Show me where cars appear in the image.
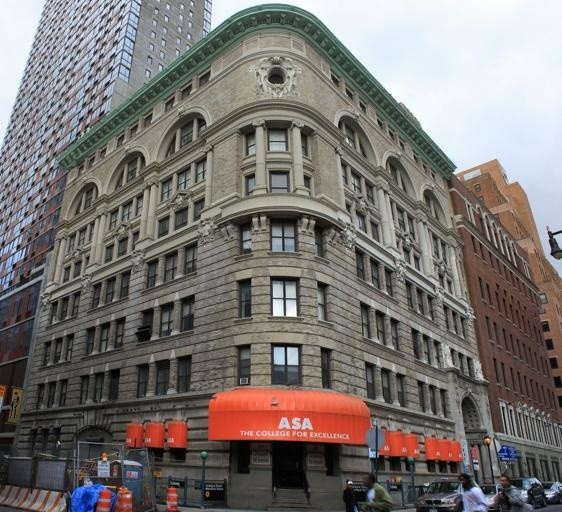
[415,477,562,512]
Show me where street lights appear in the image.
[483,435,495,484]
[545,226,562,260]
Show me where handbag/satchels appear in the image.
[520,503,533,512]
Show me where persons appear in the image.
[343,481,360,512]
[362,473,393,512]
[458,473,490,512]
[491,475,525,512]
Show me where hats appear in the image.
[347,481,353,485]
